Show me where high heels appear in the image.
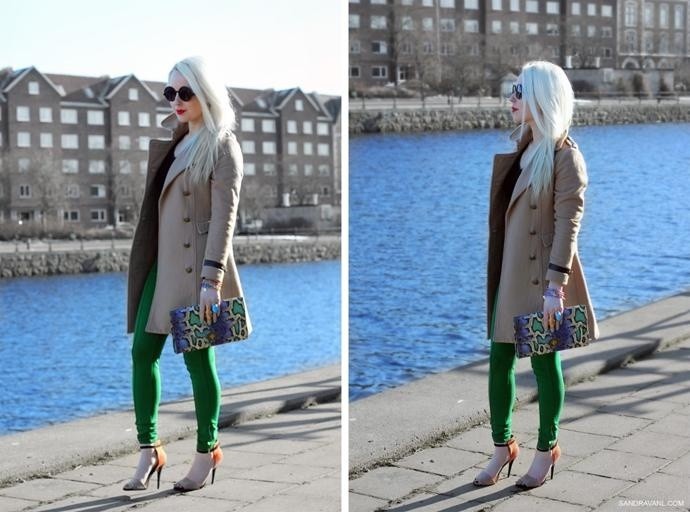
[515,439,561,491]
[173,440,224,492]
[472,435,519,487]
[123,439,168,490]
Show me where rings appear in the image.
[554,309,563,323]
[212,303,222,314]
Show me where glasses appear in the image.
[512,84,523,99]
[163,86,195,102]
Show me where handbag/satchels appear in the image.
[168,297,252,355]
[513,305,590,358]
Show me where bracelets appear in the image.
[196,280,222,289]
[542,285,568,298]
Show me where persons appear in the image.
[469,59,602,490]
[123,52,257,495]
